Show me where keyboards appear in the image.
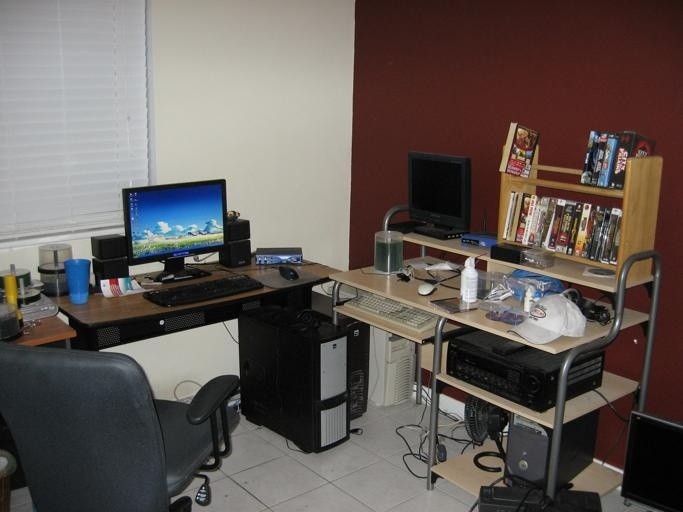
[143,273,264,308]
[343,292,438,334]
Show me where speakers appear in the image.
[90,234,130,282]
[219,216,252,269]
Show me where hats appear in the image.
[510,294,585,345]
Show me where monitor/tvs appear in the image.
[621,409,683,511]
[408,149,471,241]
[121,177,227,282]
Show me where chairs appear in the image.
[0,342,240,512]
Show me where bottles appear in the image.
[374,231,404,272]
[523,288,534,312]
[460,256,477,304]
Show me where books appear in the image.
[21,293,58,321]
[499,122,656,266]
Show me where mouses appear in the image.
[280,266,300,281]
[416,281,436,297]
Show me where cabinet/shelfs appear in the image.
[497,145,663,288]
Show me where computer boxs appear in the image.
[503,410,600,488]
[238,312,350,453]
[369,325,417,407]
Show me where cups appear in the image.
[64,259,91,305]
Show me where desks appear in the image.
[0,316,78,350]
[329,204,662,507]
[46,258,341,427]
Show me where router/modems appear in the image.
[461,232,496,249]
